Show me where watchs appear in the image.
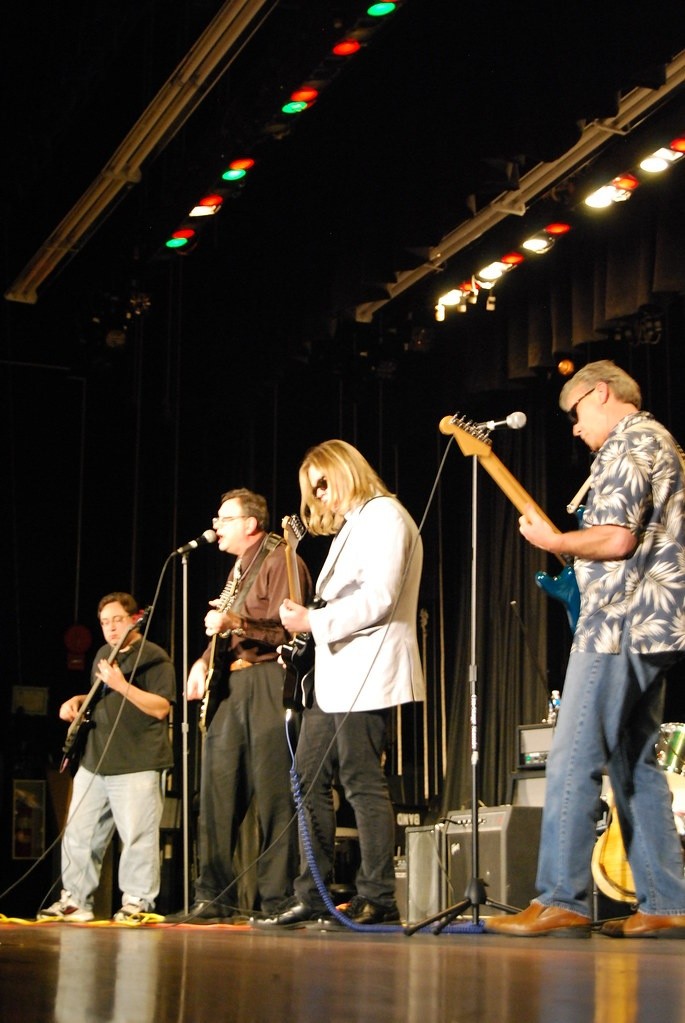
[232,616,244,636]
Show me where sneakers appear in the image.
[36,889,95,923]
[113,899,154,923]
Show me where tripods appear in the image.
[402,451,522,937]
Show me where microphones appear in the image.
[171,530,217,556]
[475,412,526,430]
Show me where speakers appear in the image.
[401,770,633,926]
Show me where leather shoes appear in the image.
[248,895,333,930]
[598,909,685,939]
[484,900,593,939]
[317,895,401,932]
[166,900,240,925]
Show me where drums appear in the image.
[655,721,685,816]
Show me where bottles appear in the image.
[547,690,560,724]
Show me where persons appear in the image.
[483,359,685,943]
[36,592,175,922]
[279,440,424,922]
[176,489,313,921]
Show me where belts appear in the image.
[223,659,261,671]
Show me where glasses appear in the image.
[99,615,129,626]
[212,514,250,525]
[567,380,611,425]
[311,475,327,498]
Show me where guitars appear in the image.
[199,576,239,729]
[280,511,328,712]
[57,603,154,779]
[439,410,588,636]
